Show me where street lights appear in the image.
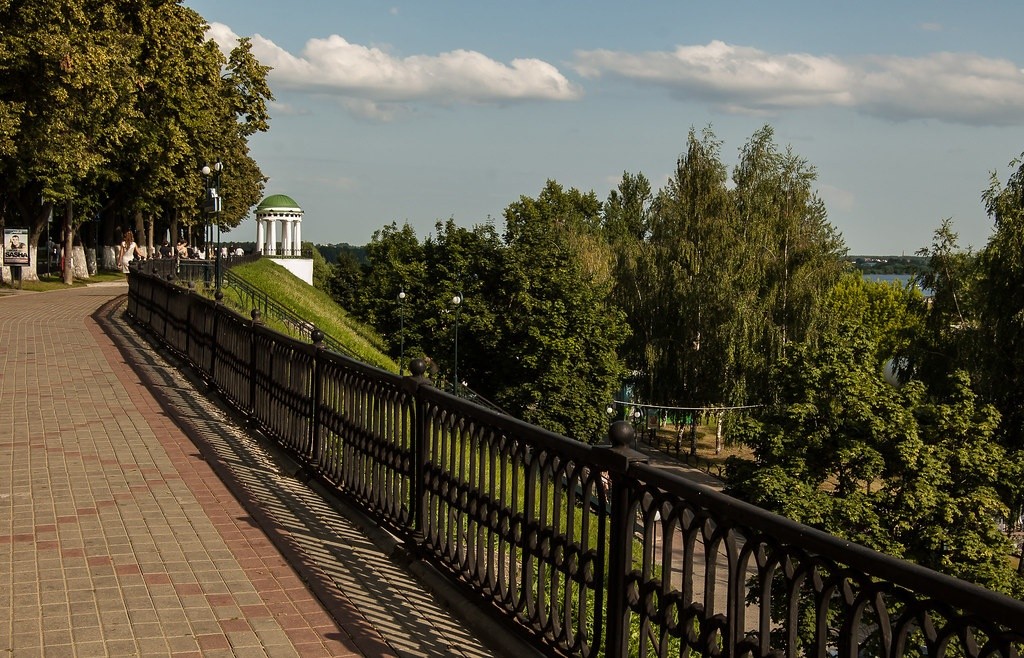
[203,165,212,287]
[451,296,464,399]
[214,161,225,293]
[635,411,640,453]
[607,407,613,445]
[399,291,406,377]
